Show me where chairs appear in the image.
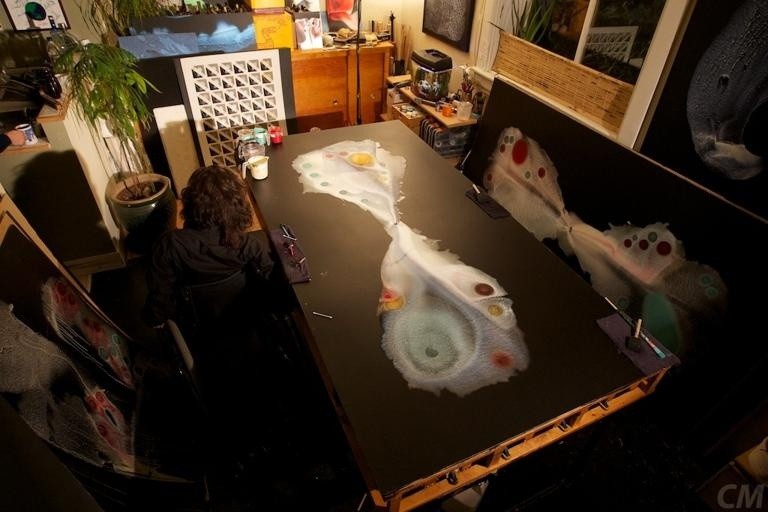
[185,270,254,335]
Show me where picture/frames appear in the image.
[420,0,475,52]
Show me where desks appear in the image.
[237,120,682,511]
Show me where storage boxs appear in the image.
[409,49,452,102]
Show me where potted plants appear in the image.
[41,24,180,253]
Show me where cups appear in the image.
[442,102,455,116]
[456,100,473,120]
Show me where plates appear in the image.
[344,150,387,173]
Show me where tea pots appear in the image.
[234,128,256,146]
[242,155,270,180]
[237,141,265,162]
[254,127,271,147]
[267,120,282,144]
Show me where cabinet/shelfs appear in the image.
[291,43,394,133]
[381,76,480,167]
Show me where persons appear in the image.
[122,164,274,336]
